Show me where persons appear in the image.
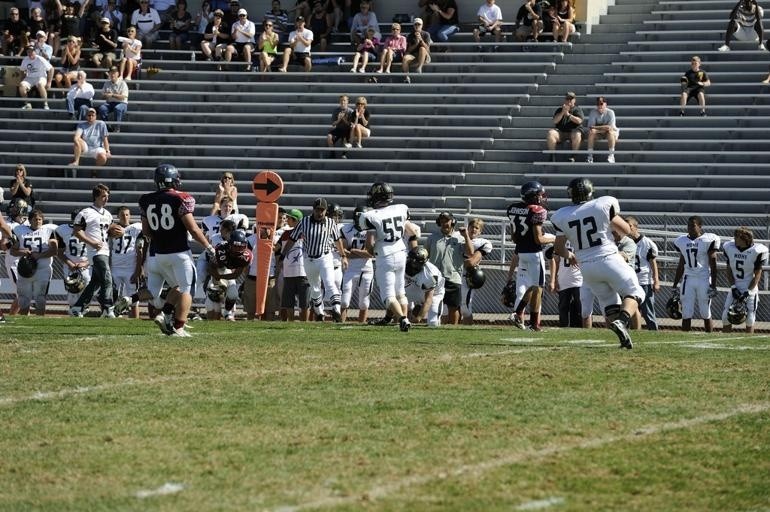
[325,94,352,147]
[553,176,649,349]
[584,97,619,163]
[211,169,240,218]
[176,0,580,73]
[716,0,767,53]
[0,0,179,134]
[347,97,373,148]
[0,180,769,334]
[67,107,113,168]
[137,161,222,338]
[545,91,584,162]
[7,163,37,217]
[678,55,711,118]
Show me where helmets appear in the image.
[17,255,38,278]
[7,198,28,221]
[228,232,247,258]
[406,246,430,277]
[284,209,303,221]
[667,295,683,320]
[465,266,487,289]
[65,273,84,293]
[568,178,595,206]
[498,281,517,307]
[520,181,545,206]
[325,202,344,223]
[727,300,747,324]
[154,165,181,190]
[368,182,393,210]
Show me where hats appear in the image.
[296,16,304,22]
[238,8,247,15]
[565,92,576,98]
[440,211,451,220]
[36,30,46,38]
[414,18,422,25]
[88,108,95,113]
[101,17,109,24]
[313,198,328,210]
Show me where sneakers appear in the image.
[376,315,411,331]
[717,45,730,51]
[67,297,238,339]
[610,320,632,350]
[475,45,503,52]
[22,103,33,109]
[514,317,545,332]
[316,309,342,322]
[606,155,614,162]
[758,44,767,50]
[43,103,49,109]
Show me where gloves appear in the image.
[733,287,750,301]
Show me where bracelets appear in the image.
[106,151,110,153]
[567,113,572,123]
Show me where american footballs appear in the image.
[108,221,123,237]
